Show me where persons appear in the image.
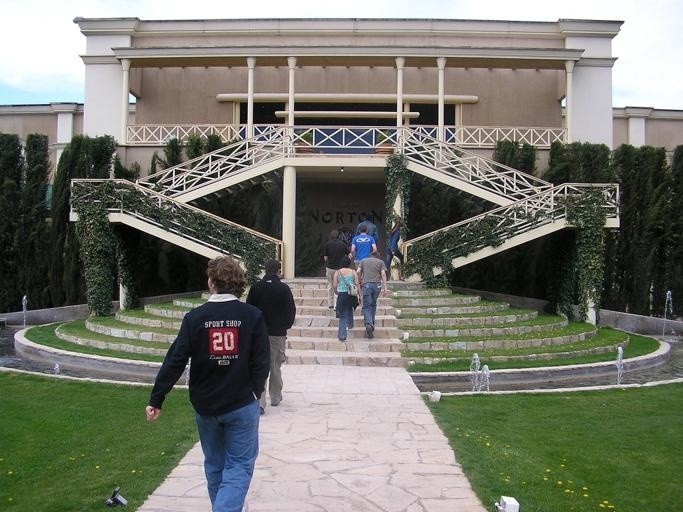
[322,211,409,342]
[246,259,298,413]
[144,257,273,510]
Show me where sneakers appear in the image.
[366,325,374,338]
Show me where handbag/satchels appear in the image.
[347,284,358,296]
